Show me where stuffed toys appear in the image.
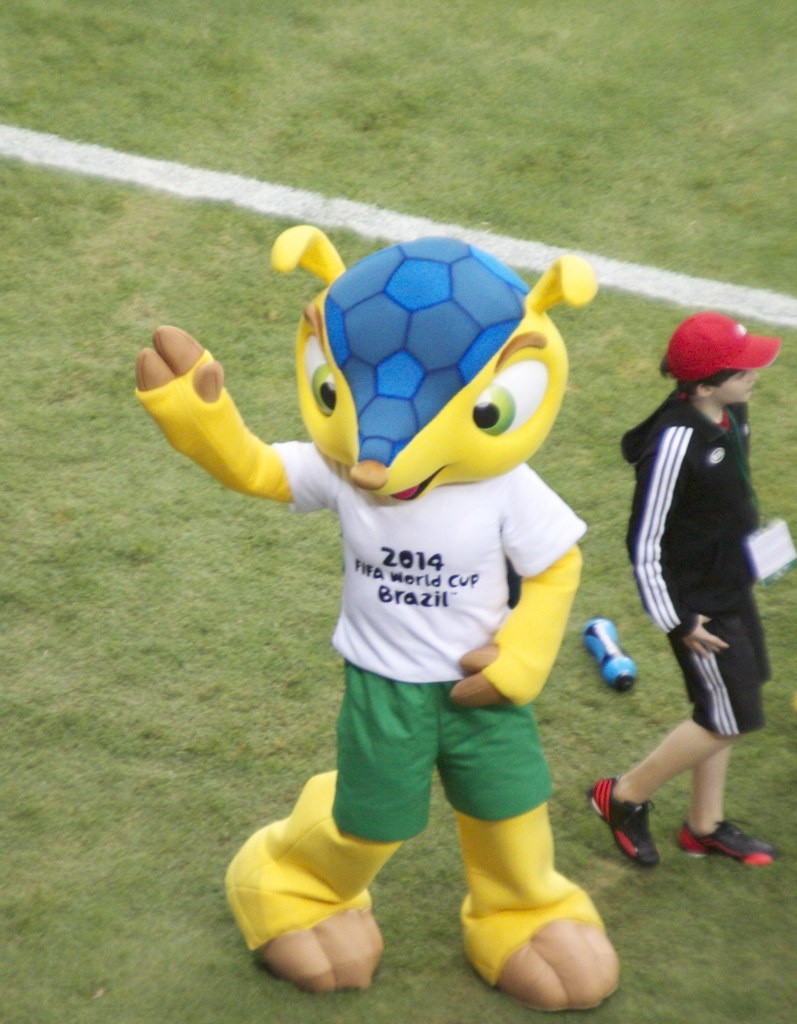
[131,225,619,1011]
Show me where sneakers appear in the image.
[586,774,661,867]
[676,819,781,866]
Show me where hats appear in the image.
[667,311,782,382]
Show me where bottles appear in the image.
[581,617,636,692]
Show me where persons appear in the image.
[583,312,783,870]
